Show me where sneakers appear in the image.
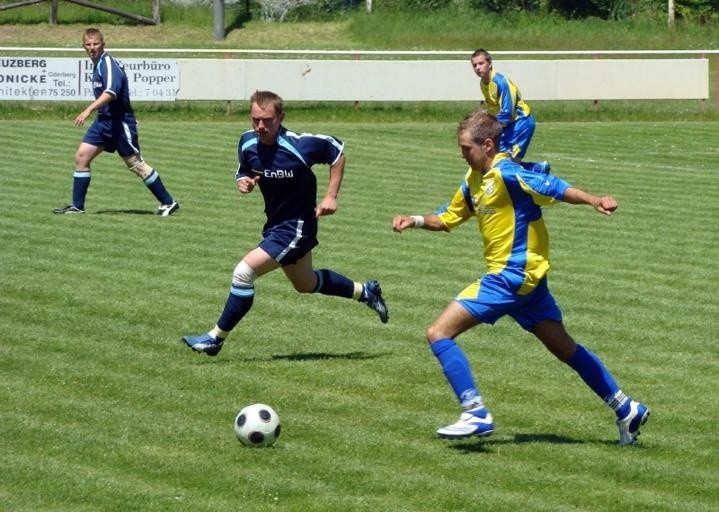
[53,205,84,214]
[182,333,224,355]
[436,405,494,436]
[364,280,387,323]
[156,200,178,216]
[616,400,650,447]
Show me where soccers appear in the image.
[233,404,280,448]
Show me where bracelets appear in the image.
[410,214,427,230]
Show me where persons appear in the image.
[52,27,181,219]
[388,112,652,449]
[181,88,389,355]
[471,46,536,164]
